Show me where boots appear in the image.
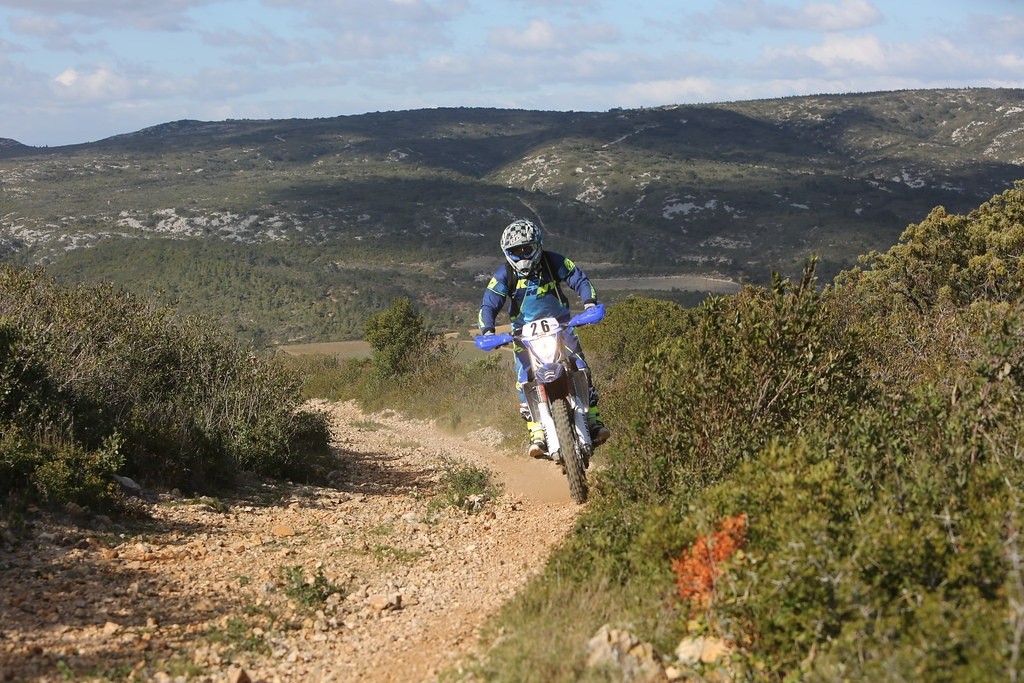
[587,406,610,442]
[527,421,548,459]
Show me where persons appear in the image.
[477,220,611,459]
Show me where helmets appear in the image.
[500,220,543,277]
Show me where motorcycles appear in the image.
[474,304,607,505]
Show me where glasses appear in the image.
[504,242,537,260]
